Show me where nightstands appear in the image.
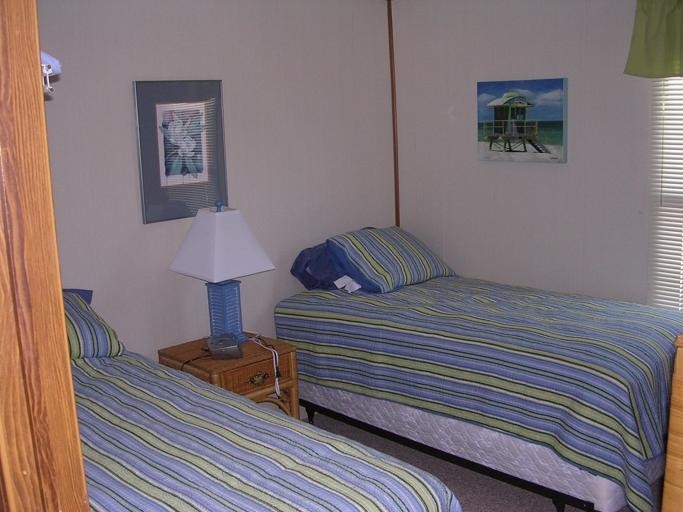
[154,327,304,422]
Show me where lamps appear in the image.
[165,199,278,352]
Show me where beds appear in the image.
[48,281,476,512]
[261,263,681,511]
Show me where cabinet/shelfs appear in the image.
[652,329,681,511]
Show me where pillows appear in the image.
[324,224,457,296]
[57,286,132,365]
[285,224,382,293]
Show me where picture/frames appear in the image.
[130,76,231,227]
